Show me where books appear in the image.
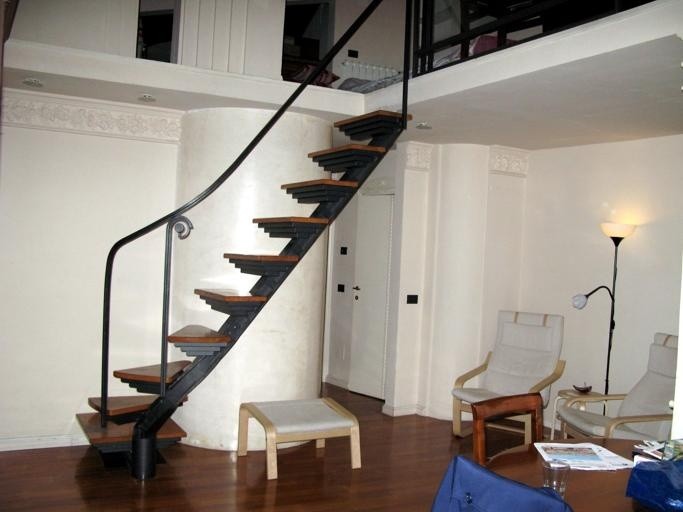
[532,438,682,472]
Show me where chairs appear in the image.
[469,392,544,467]
[556,331,677,442]
[451,311,567,445]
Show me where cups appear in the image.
[540,460,571,500]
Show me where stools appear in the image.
[236,397,363,481]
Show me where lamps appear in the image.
[571,223,636,416]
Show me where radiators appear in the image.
[342,61,399,83]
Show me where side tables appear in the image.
[549,388,609,442]
